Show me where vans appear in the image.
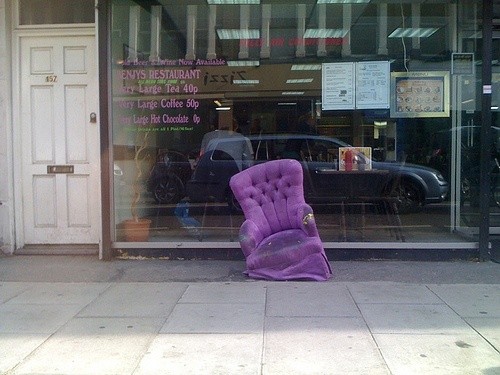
[189,133,447,215]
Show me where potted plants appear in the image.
[122,52,180,242]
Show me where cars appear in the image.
[142,143,203,204]
[114,164,127,191]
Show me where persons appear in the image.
[297,110,320,136]
[200,117,255,210]
[345,148,366,172]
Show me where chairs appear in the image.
[148,149,240,242]
[230,159,333,281]
[360,149,407,243]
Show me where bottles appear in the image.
[345,148,352,172]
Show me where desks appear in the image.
[315,166,389,242]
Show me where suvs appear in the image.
[433,124,499,206]
